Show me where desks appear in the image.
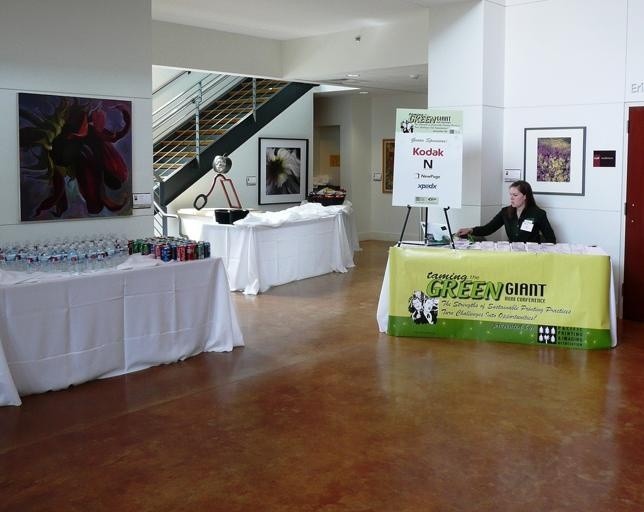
[0,254,244,410]
[376,241,617,351]
[180,206,363,295]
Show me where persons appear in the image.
[455,181,556,245]
[411,291,437,325]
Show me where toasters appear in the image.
[1,233,130,276]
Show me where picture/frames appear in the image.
[382,138,395,194]
[523,128,586,196]
[258,137,309,205]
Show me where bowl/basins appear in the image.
[451,239,591,253]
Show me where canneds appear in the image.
[128,235,211,262]
